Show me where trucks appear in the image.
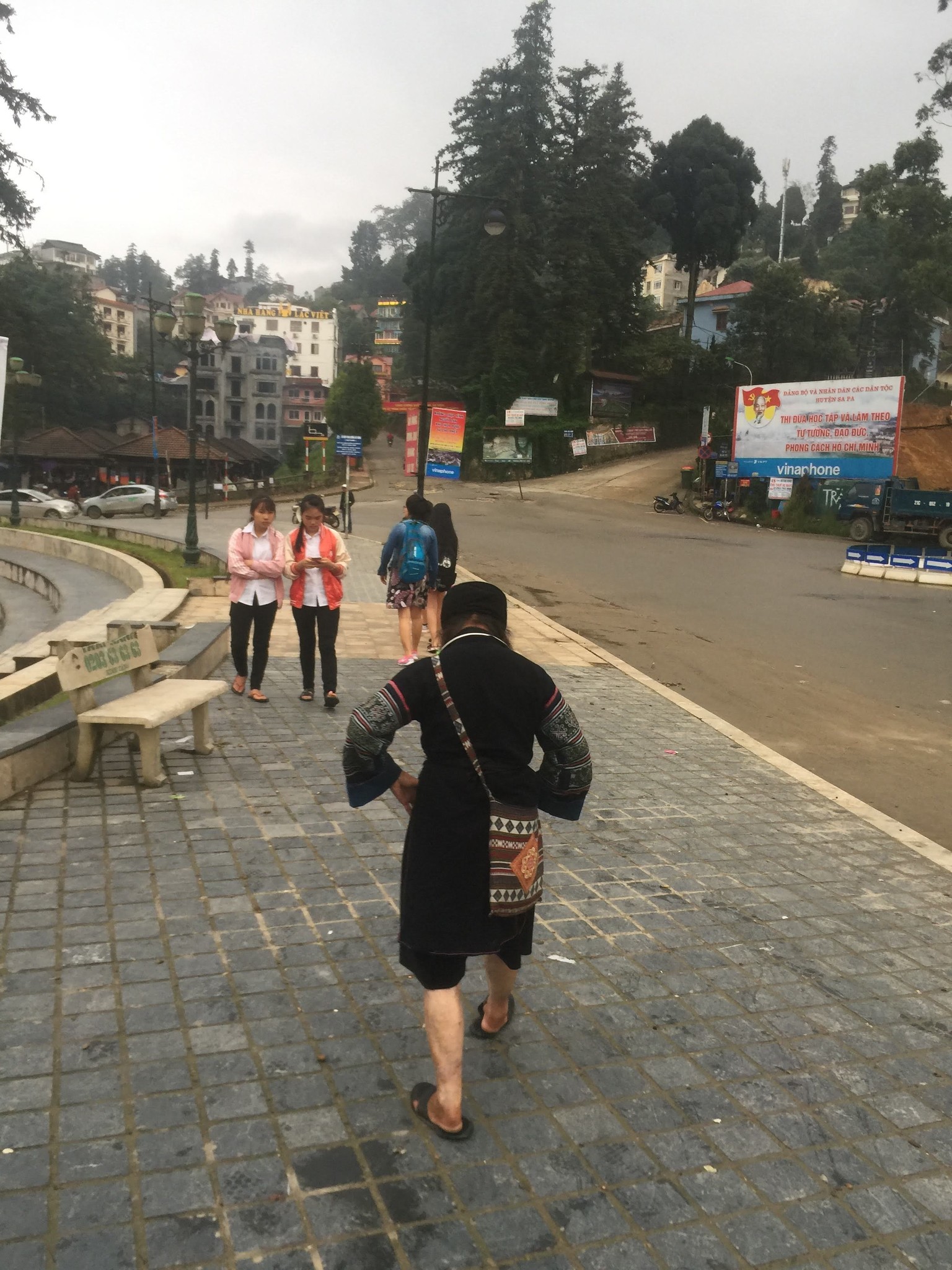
[835,475,952,551]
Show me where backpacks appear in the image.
[399,521,426,582]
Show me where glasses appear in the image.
[403,506,407,509]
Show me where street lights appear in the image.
[778,157,791,264]
[725,356,753,386]
[407,157,512,496]
[154,292,238,565]
[141,280,178,520]
[4,357,42,526]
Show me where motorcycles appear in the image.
[652,492,685,514]
[702,498,731,522]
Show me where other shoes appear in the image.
[348,531,352,533]
[341,530,345,532]
[426,643,441,654]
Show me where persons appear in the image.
[748,394,771,428]
[283,494,352,707]
[68,485,82,509]
[386,433,394,445]
[421,500,457,653]
[341,582,593,1142]
[228,495,285,703]
[377,493,438,665]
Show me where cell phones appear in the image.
[312,557,322,563]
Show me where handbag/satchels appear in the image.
[488,800,544,916]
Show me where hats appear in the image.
[342,484,347,488]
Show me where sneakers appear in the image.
[421,623,430,633]
[411,650,418,660]
[398,655,414,665]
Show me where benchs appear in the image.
[56,622,228,783]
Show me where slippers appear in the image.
[409,1082,473,1139]
[299,688,315,701]
[232,674,248,695]
[248,693,269,703]
[324,694,339,708]
[469,992,514,1039]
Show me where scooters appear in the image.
[64,490,80,503]
[388,437,394,447]
[292,501,302,525]
[320,494,340,528]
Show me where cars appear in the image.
[82,484,179,520]
[0,488,80,520]
